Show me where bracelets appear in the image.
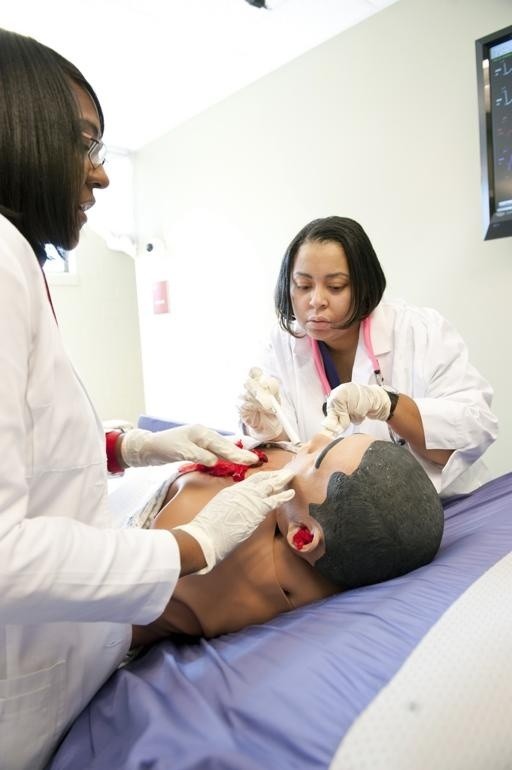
[104,424,127,474]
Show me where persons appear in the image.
[0,24,318,770]
[97,431,445,647]
[233,215,499,500]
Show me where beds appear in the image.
[49,417,511,769]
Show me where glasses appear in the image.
[80,129,105,170]
